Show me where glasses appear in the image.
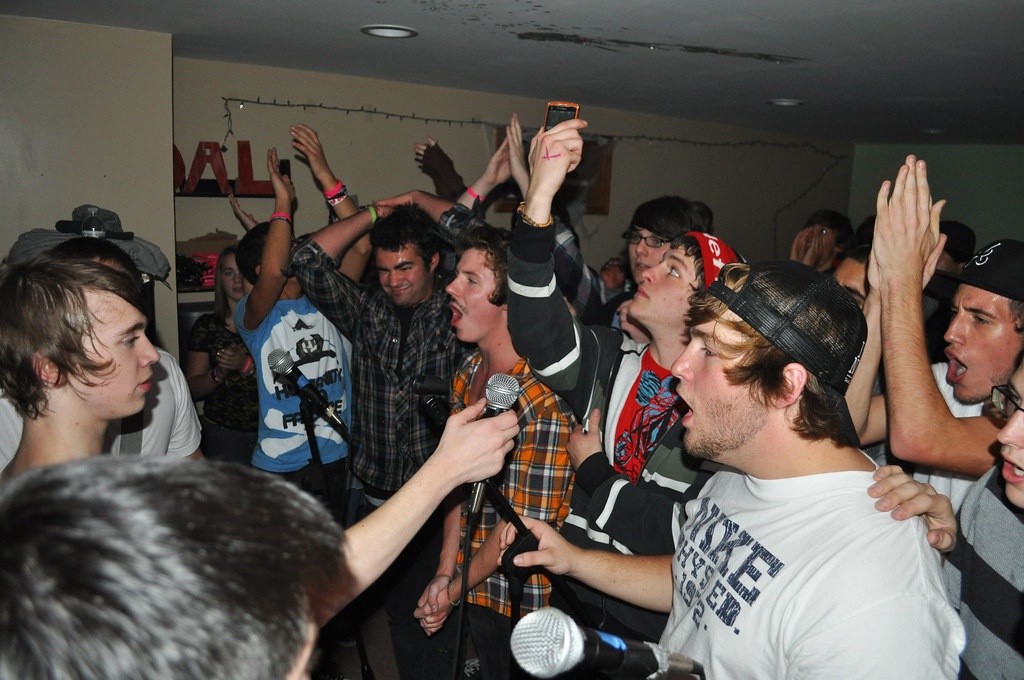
[991,384,1023,418]
[621,231,673,248]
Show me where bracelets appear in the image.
[245,367,256,377]
[210,367,224,383]
[322,179,342,198]
[447,577,461,606]
[367,206,376,224]
[328,195,347,207]
[466,186,486,202]
[208,369,217,384]
[329,184,347,199]
[270,212,291,223]
[516,202,553,227]
[242,356,253,373]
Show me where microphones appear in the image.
[269,347,348,434]
[510,606,704,678]
[468,373,520,527]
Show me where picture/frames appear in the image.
[490,124,613,216]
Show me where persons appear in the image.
[0,111,1024,678]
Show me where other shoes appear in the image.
[338,631,357,648]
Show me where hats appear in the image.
[938,219,976,258]
[941,239,1024,299]
[685,231,742,289]
[707,258,868,446]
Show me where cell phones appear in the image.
[543,102,580,134]
